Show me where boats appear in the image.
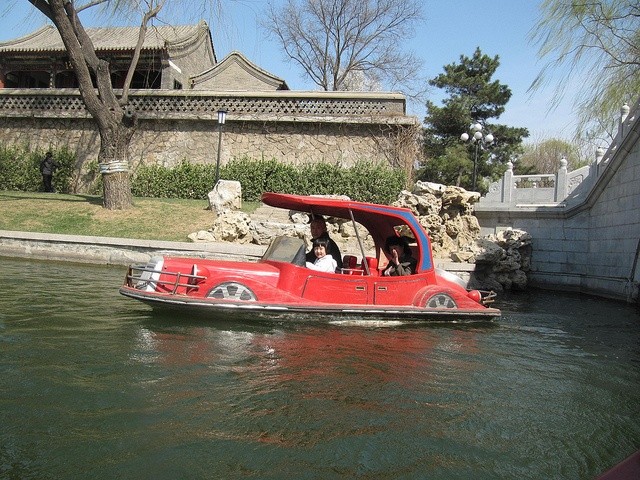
[119,192,502,318]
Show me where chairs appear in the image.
[409,246,419,261]
[336,255,361,274]
[353,256,379,276]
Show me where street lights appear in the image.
[216,108,228,184]
[460,123,494,191]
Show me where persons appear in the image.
[382,235,417,276]
[306,238,338,273]
[41,152,57,192]
[305,217,343,273]
